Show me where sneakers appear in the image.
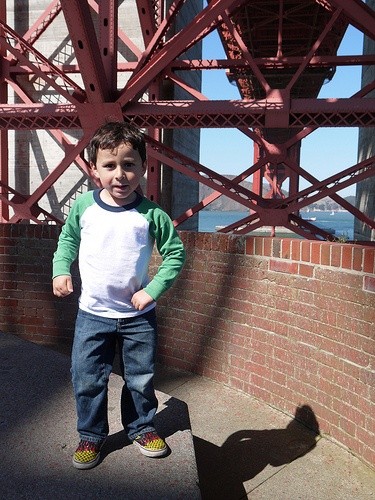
[72,436,108,469]
[132,431,168,457]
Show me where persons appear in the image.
[53,122,186,470]
